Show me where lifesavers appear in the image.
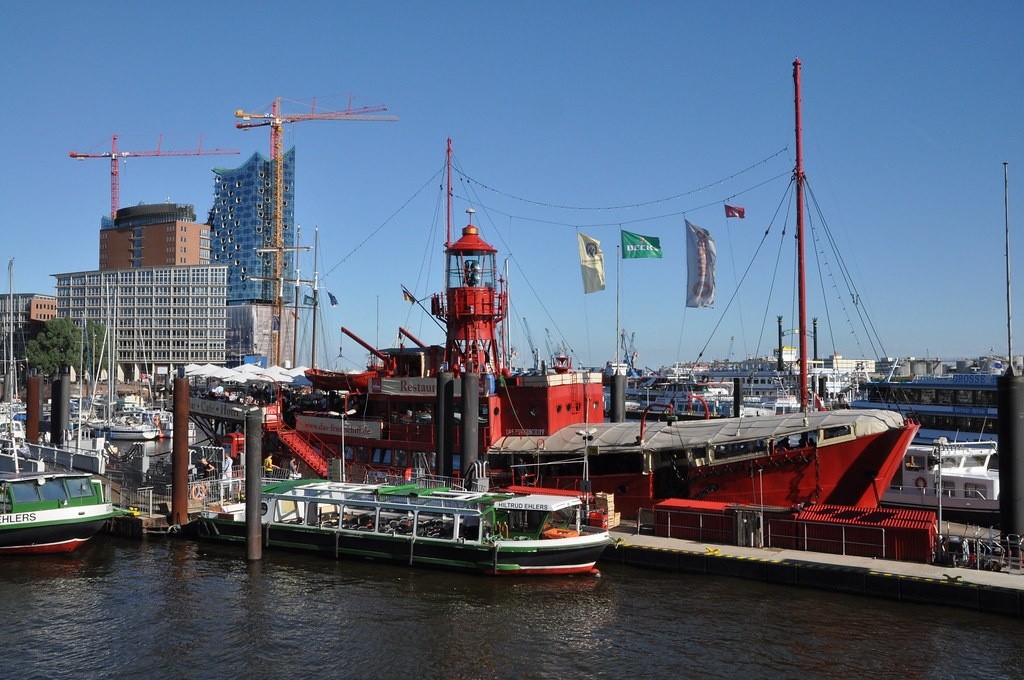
[915,477,927,490]
[155,461,165,475]
[191,484,207,500]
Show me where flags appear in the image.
[621,230,662,259]
[725,205,745,219]
[685,219,716,310]
[578,232,605,295]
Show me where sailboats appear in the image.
[0,58,1003,579]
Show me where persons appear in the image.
[225,452,233,500]
[264,454,273,472]
[289,457,297,474]
[207,377,340,412]
[201,457,214,478]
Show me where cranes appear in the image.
[233,92,400,363]
[68,131,240,220]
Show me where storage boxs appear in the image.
[579,492,622,532]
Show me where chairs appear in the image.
[323,511,444,537]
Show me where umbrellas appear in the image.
[170,362,362,386]
